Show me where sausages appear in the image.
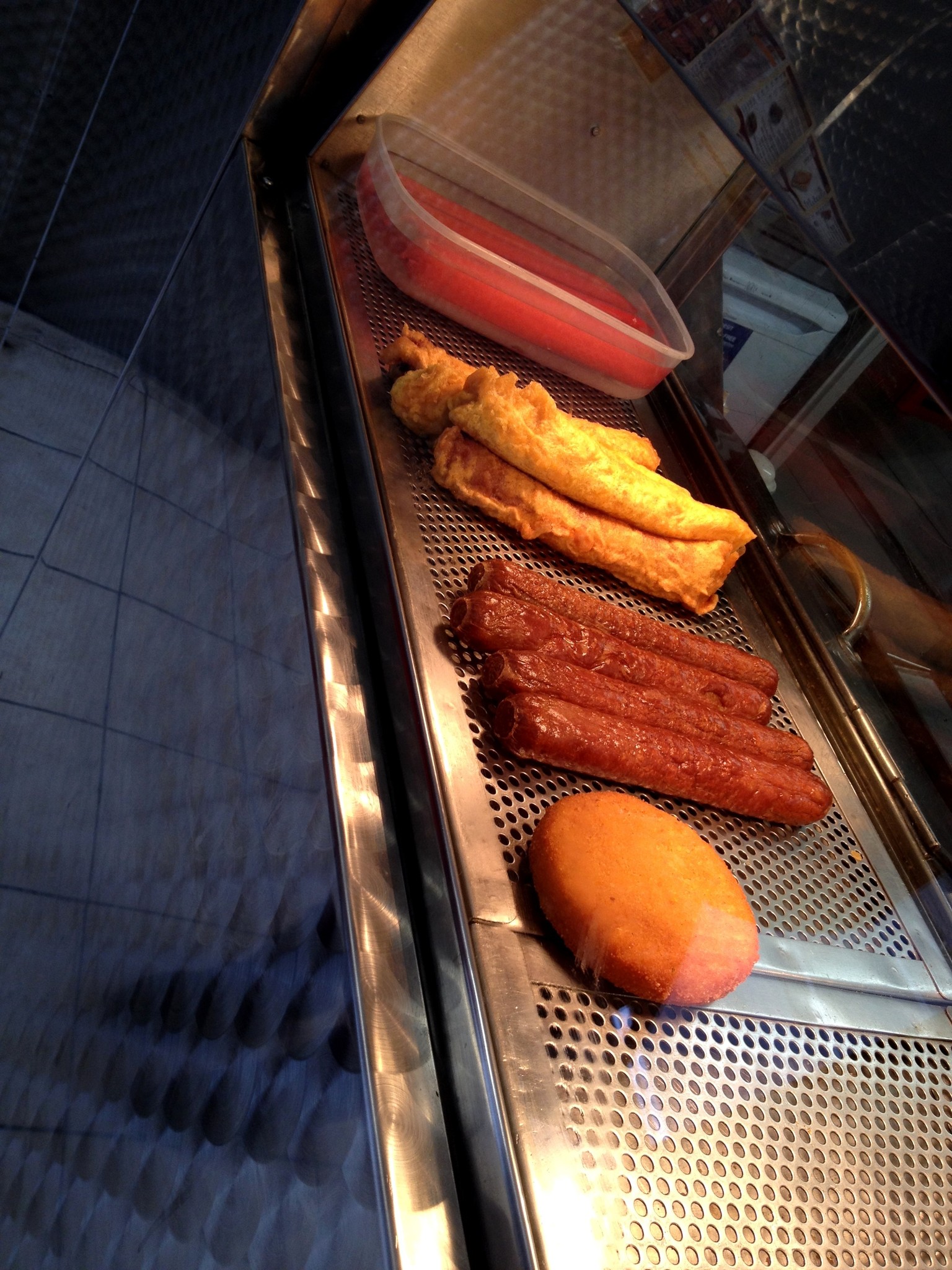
[448,557,835,829]
[374,162,670,386]
